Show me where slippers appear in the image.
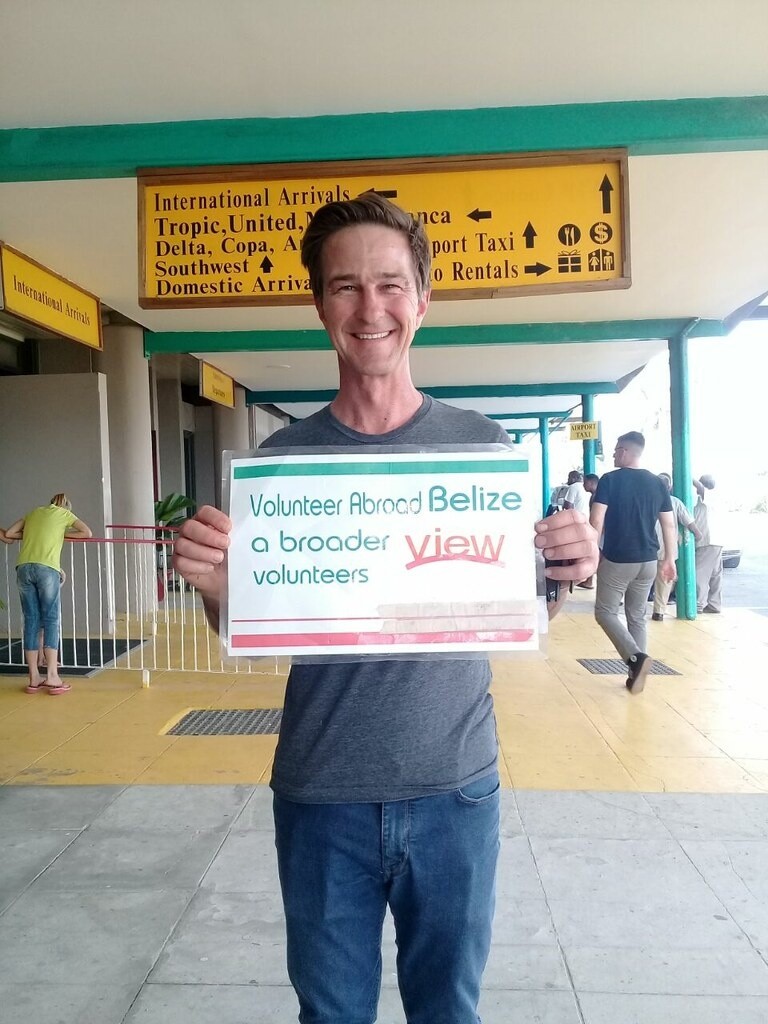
[577,582,594,589]
[25,680,47,693]
[49,681,73,695]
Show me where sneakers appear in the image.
[625,652,651,696]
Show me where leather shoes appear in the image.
[651,611,664,621]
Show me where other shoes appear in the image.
[696,605,722,614]
[666,600,676,605]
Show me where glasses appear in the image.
[545,504,574,603]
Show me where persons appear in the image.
[562,471,593,523]
[588,432,677,694]
[0,493,93,695]
[693,474,725,614]
[576,474,604,589]
[647,473,703,621]
[172,191,599,1024]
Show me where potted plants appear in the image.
[153,492,199,602]
